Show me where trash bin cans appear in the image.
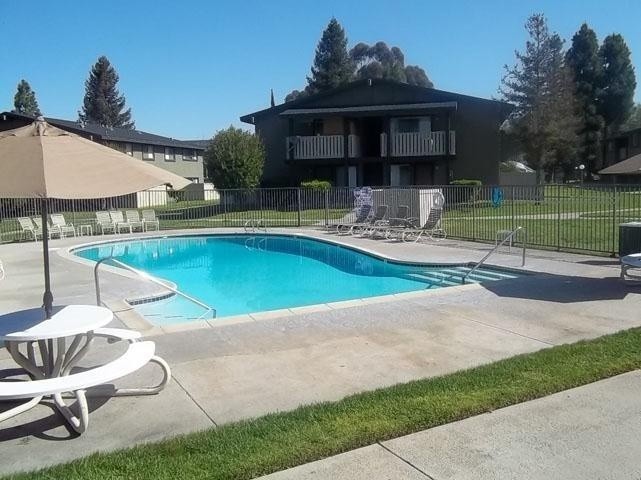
[619,222,641,257]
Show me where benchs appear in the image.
[0,304,172,435]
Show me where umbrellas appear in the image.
[0,114,195,319]
[595,150,641,176]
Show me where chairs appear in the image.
[94,208,161,234]
[325,203,448,243]
[17,213,76,242]
[620,252,641,286]
